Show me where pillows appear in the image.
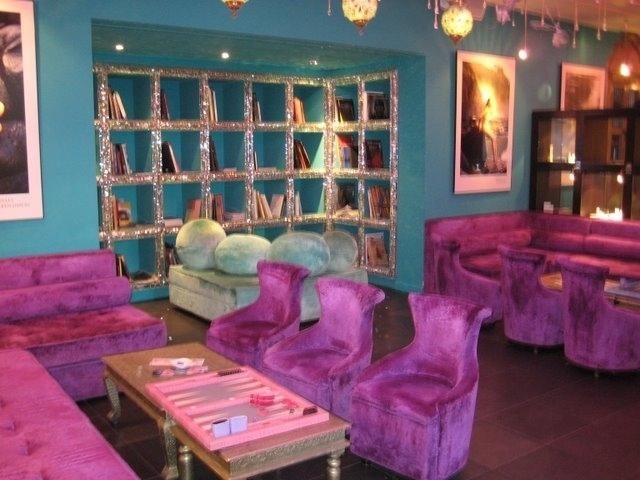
[175,218,360,282]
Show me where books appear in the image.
[364,91,386,266]
[337,97,358,217]
[109,91,130,282]
[294,98,311,218]
[208,88,243,223]
[251,92,284,221]
[159,89,201,272]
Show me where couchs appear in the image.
[425,210,639,294]
[0,250,167,480]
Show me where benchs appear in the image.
[161,226,372,329]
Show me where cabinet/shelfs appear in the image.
[529,109,639,222]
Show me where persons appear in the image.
[477,97,502,172]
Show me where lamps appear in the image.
[338,0,474,46]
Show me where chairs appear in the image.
[432,231,639,376]
